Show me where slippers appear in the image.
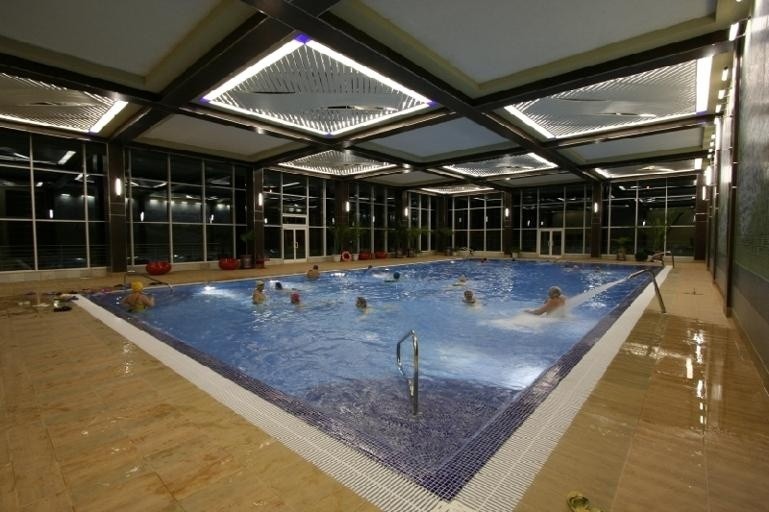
[567,491,605,512]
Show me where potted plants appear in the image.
[510,249,521,257]
[240,232,254,269]
[642,214,668,261]
[332,219,369,262]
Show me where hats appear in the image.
[393,271,400,279]
[291,292,300,300]
[256,280,265,287]
[313,265,318,269]
[358,296,367,306]
[463,291,473,295]
[131,282,143,291]
[549,286,561,296]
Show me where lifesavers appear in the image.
[341,251,353,262]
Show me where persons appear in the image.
[120,280,155,314]
[454,274,469,286]
[461,289,476,303]
[304,264,321,280]
[355,296,369,314]
[290,292,301,304]
[274,282,282,290]
[252,279,266,304]
[524,286,567,318]
